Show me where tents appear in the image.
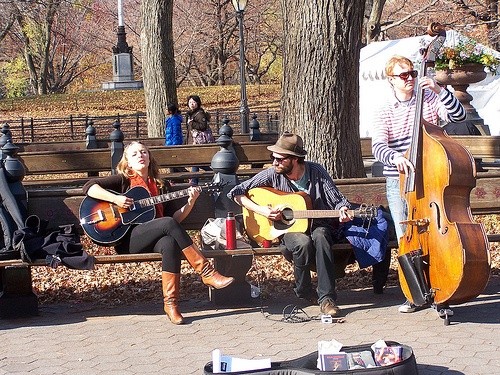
[360,28,500,137]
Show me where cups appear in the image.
[263,240,272,248]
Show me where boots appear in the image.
[162,271,185,324]
[182,242,235,290]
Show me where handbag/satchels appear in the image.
[200,216,243,247]
[190,111,215,144]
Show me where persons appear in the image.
[164,103,189,183]
[83,140,235,324]
[186,95,217,184]
[227,131,353,317]
[371,56,467,313]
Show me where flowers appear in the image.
[422,35,500,76]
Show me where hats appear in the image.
[267,130,308,158]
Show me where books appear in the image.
[320,347,401,370]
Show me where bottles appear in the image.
[226,212,237,250]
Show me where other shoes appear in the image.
[320,299,340,316]
[398,300,419,313]
[308,289,318,305]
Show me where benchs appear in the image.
[0,138,500,304]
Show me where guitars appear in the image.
[79,182,224,248]
[242,186,378,248]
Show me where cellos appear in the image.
[397,21,493,327]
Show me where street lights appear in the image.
[231,0,249,133]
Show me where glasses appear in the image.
[270,154,291,162]
[165,108,168,111]
[388,70,418,79]
